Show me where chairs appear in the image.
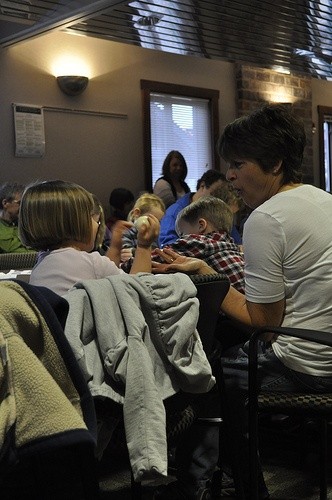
[0,253,38,273]
[239,326,332,500]
[167,273,231,500]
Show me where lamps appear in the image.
[57,54,88,97]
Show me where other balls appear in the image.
[135,216,150,232]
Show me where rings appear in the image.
[168,257,175,264]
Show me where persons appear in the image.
[150,103,332,500]
[0,151,255,347]
